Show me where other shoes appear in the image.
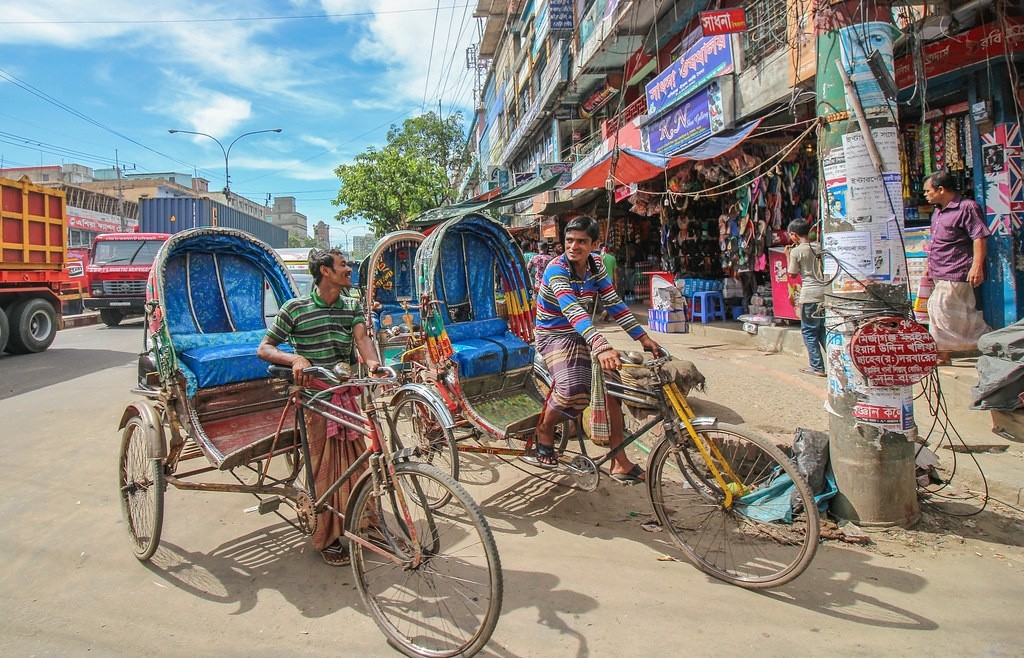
[599,318,609,324]
[936,357,953,366]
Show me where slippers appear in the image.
[800,368,825,377]
[659,142,820,277]
[369,527,403,551]
[536,434,558,465]
[613,463,646,480]
[321,538,350,566]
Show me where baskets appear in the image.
[616,352,693,409]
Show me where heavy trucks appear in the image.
[81,194,289,324]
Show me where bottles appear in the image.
[624,261,653,304]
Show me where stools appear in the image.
[691,291,726,326]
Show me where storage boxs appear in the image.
[648,309,686,334]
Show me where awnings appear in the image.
[406,113,764,228]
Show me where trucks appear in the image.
[0,174,72,357]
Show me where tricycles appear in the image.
[387,211,822,591]
[119,225,506,656]
[360,231,428,380]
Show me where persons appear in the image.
[534,215,665,484]
[923,172,996,366]
[737,265,760,315]
[256,246,395,567]
[519,240,619,324]
[784,218,828,377]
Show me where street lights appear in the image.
[166,128,283,190]
[326,225,365,253]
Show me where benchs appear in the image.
[367,229,426,366]
[411,208,539,403]
[134,222,304,424]
